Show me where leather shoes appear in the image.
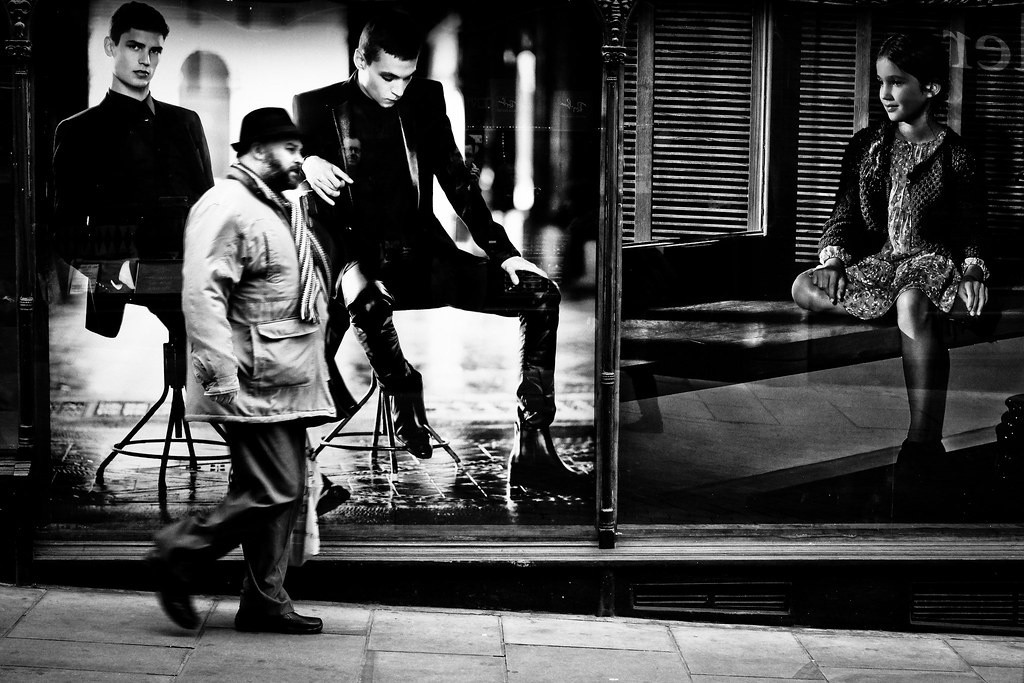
[142,548,203,630]
[389,381,433,459]
[509,421,583,498]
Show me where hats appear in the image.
[231,107,307,152]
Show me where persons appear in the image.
[293,25,588,489]
[791,32,990,476]
[46,2,350,516]
[146,107,335,634]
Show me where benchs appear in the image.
[618,286,1024,401]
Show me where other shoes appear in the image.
[894,438,976,507]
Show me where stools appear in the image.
[314,297,460,473]
[95,295,231,506]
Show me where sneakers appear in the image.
[234,606,323,634]
